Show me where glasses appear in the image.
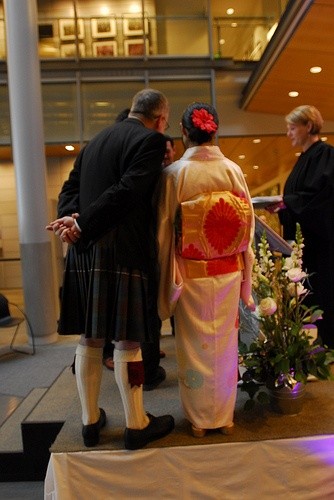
[155,114,169,130]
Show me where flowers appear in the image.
[238,221,334,412]
[191,109,219,135]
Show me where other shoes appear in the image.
[143,368,166,391]
[103,358,115,370]
[124,412,175,451]
[82,408,107,447]
[306,373,319,382]
[160,350,167,358]
[221,426,233,434]
[191,425,207,437]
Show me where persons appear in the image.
[45,89,175,451]
[277,105,334,349]
[156,102,255,438]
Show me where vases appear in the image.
[270,366,306,415]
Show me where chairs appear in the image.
[0,293,36,356]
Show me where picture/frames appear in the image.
[122,37,150,56]
[89,15,118,39]
[121,10,150,37]
[91,39,119,57]
[59,42,87,58]
[58,18,86,41]
